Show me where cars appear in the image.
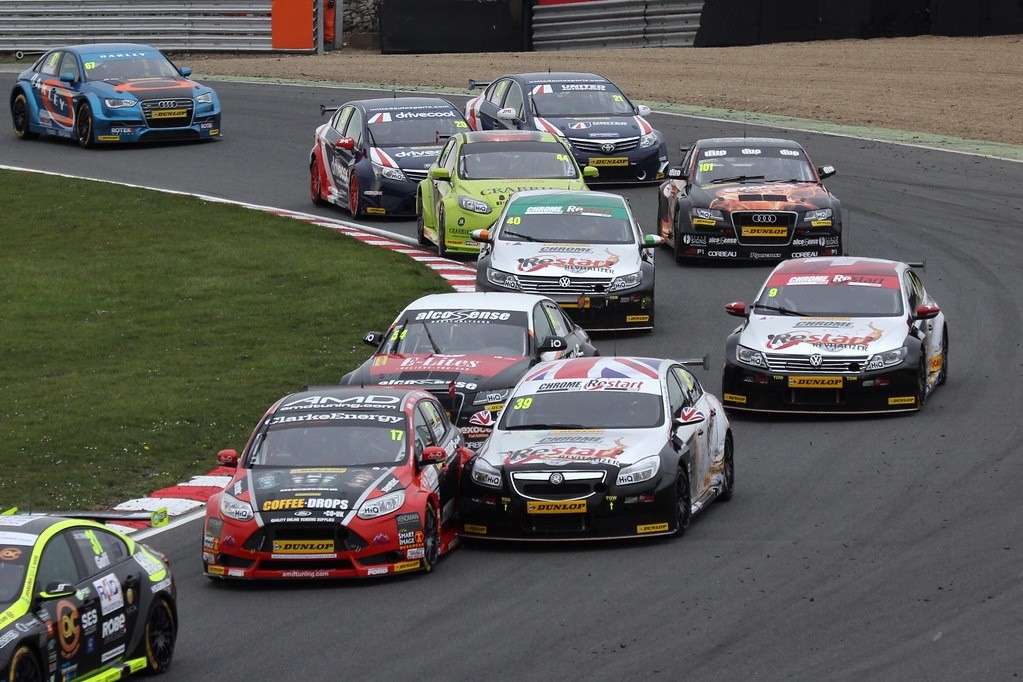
[9,43,222,148]
[309,98,481,221]
[0,507,178,681]
[468,189,666,331]
[202,386,474,579]
[415,130,601,257]
[465,71,670,184]
[454,356,736,541]
[339,292,601,451]
[721,257,948,416]
[658,138,842,268]
